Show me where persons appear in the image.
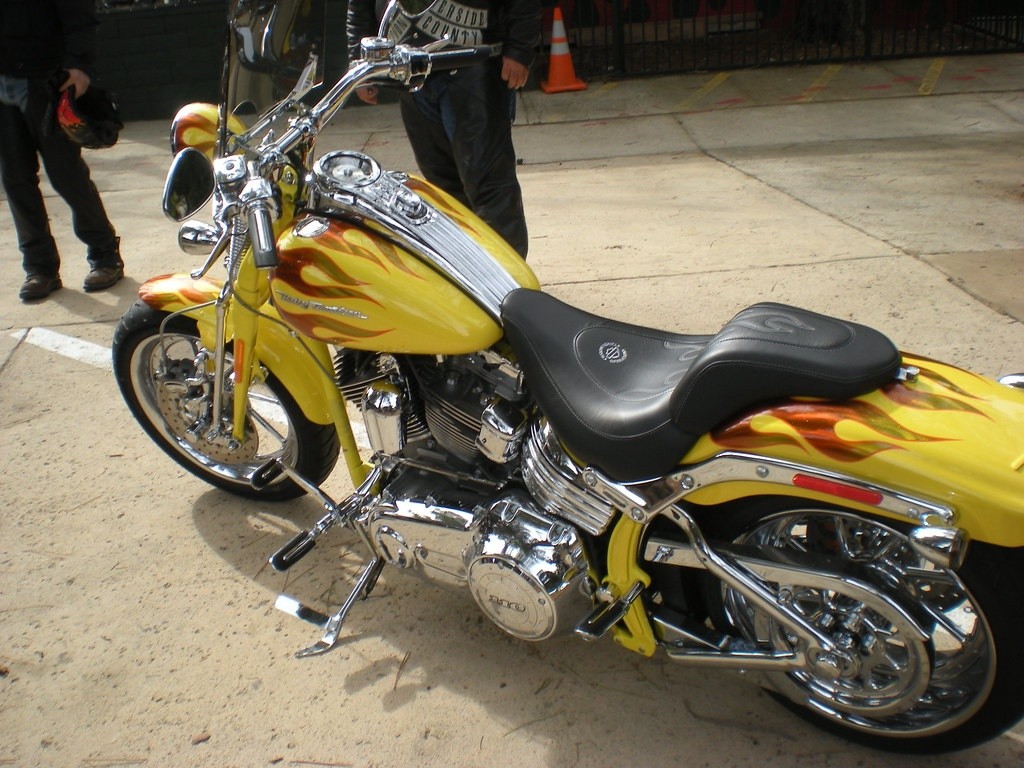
[347,0,542,262]
[1,1,124,299]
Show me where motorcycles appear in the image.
[110,0,1024,754]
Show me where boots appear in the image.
[19,273,62,300]
[83,236,124,290]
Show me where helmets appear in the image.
[57,84,124,149]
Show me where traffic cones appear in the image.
[539,5,589,95]
